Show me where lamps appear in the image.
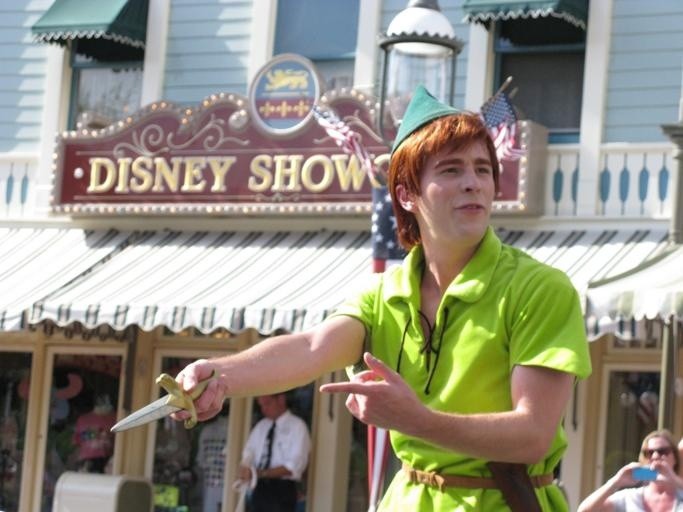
[372,0,462,155]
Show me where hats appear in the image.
[390,85,460,159]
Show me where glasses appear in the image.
[643,448,673,458]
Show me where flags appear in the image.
[307,101,361,154]
[483,94,519,162]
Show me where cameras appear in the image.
[632,465,658,481]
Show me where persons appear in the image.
[194,416,228,511]
[174,112,593,512]
[576,429,682,512]
[237,394,313,512]
[71,392,116,465]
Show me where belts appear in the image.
[402,462,555,494]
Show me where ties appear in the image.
[257,422,276,470]
